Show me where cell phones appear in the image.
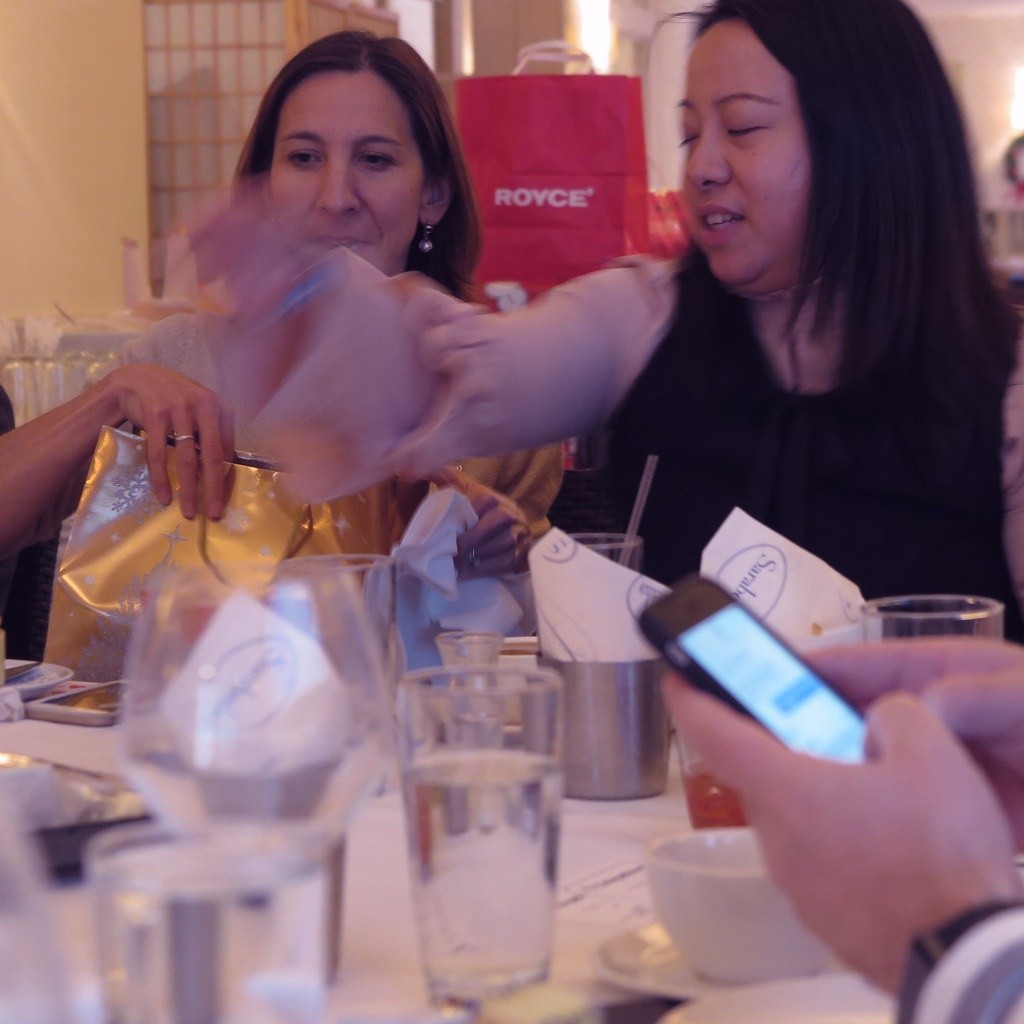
[24,680,127,727]
[639,572,866,762]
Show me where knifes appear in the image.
[5,661,42,681]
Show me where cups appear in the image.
[645,828,844,983]
[80,822,344,1023]
[671,719,750,830]
[111,568,385,824]
[539,653,670,800]
[568,533,643,571]
[432,632,505,694]
[862,596,1006,642]
[395,667,568,1000]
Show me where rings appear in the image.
[176,434,194,440]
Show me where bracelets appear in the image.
[898,899,1024,1024]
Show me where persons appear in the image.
[663,638,1024,1024]
[0,33,564,712]
[199,0,1024,644]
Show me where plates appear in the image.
[654,974,900,1024]
[591,920,693,1003]
[0,656,73,699]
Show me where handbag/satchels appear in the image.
[455,39,647,323]
